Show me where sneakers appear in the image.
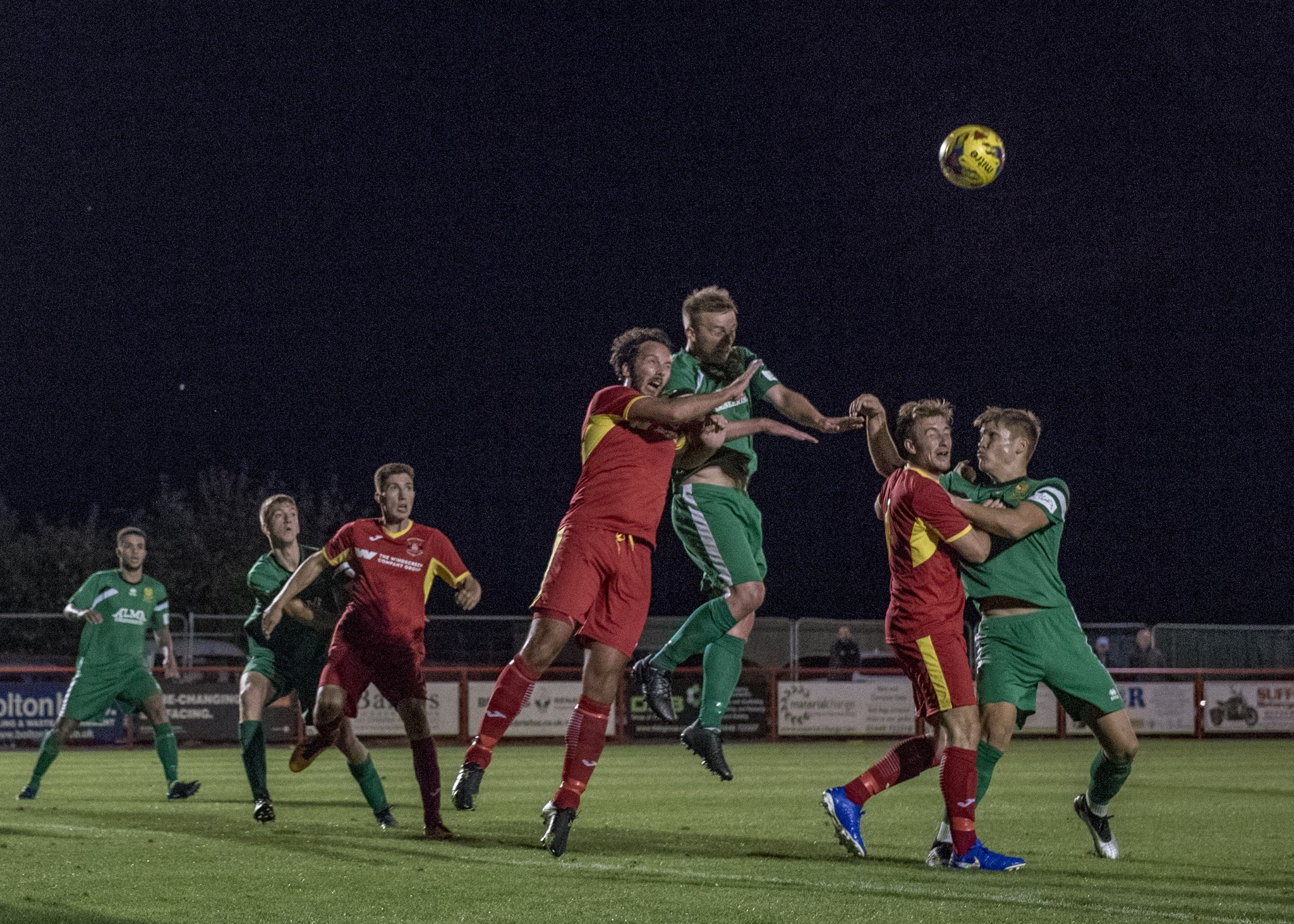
[924,840,953,870]
[629,653,678,726]
[423,822,454,841]
[541,800,580,858]
[947,837,1025,872]
[452,762,484,811]
[1073,794,1120,861]
[289,734,329,772]
[820,787,867,859]
[680,718,733,781]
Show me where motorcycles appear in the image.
[1210,684,1258,726]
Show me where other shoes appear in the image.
[375,805,398,828]
[252,800,275,823]
[166,780,201,798]
[16,786,36,802]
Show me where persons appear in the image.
[452,326,763,858]
[16,527,201,802]
[238,495,399,829]
[1126,630,1166,669]
[827,627,862,681]
[262,462,481,838]
[823,399,1025,870]
[848,393,1139,869]
[631,289,865,782]
[1092,636,1118,668]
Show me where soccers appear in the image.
[938,124,1006,189]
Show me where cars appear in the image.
[142,638,250,685]
[624,646,762,670]
[775,651,907,681]
